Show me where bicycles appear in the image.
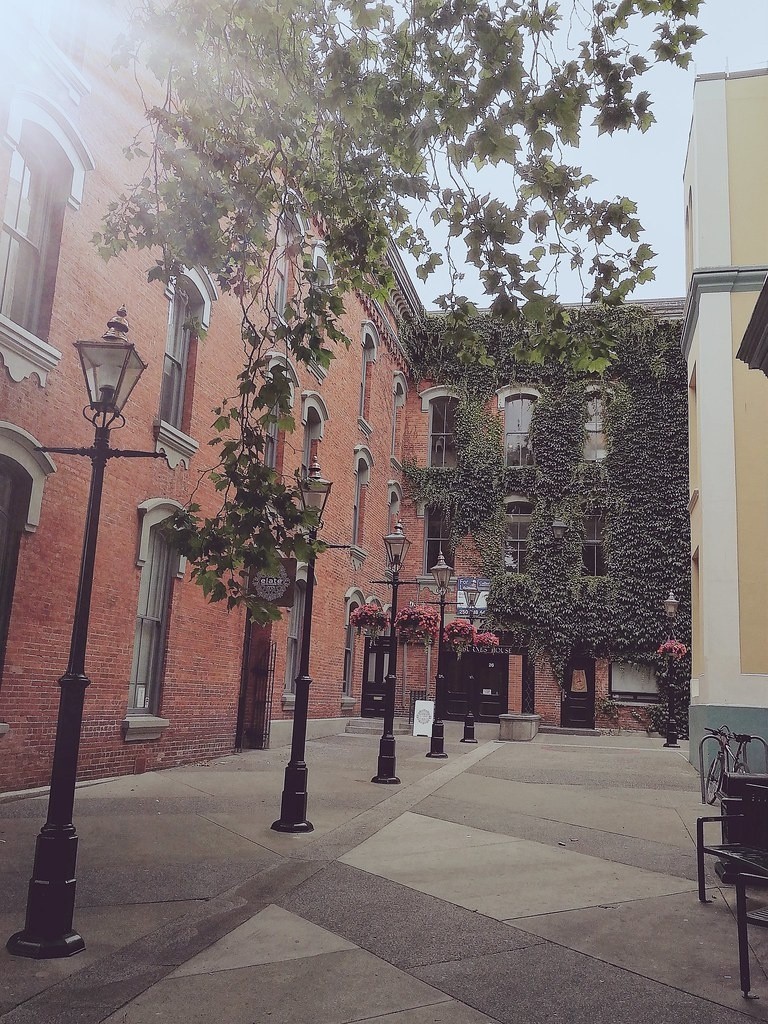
[704,724,768,804]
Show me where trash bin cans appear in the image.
[713,772,768,886]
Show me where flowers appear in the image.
[394,605,442,646]
[473,632,499,648]
[350,603,390,648]
[443,618,476,661]
[657,640,687,661]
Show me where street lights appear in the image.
[270,454,350,834]
[422,550,464,758]
[8,303,167,958]
[369,519,421,785]
[662,587,681,747]
[454,577,490,743]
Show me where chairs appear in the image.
[734,873,768,999]
[697,783,768,903]
